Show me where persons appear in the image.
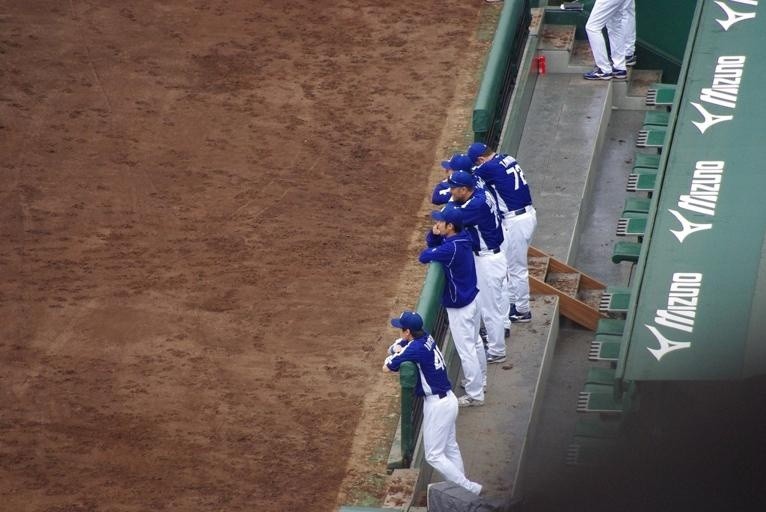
[583,0,628,80]
[467,142,537,323]
[440,170,508,364]
[419,206,487,407]
[432,154,512,339]
[609,0,637,67]
[382,310,482,496]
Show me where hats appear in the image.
[440,170,474,189]
[468,143,488,162]
[432,203,464,225]
[441,151,473,170]
[391,310,423,330]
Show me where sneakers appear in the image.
[609,54,637,65]
[612,66,627,79]
[475,248,500,256]
[458,303,532,407]
[583,68,613,80]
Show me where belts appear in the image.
[515,208,526,215]
[439,392,446,399]
